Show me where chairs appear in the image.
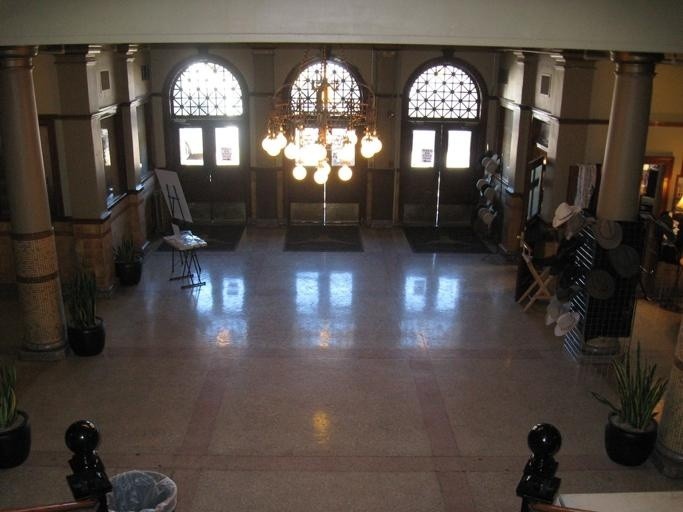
[515,231,560,315]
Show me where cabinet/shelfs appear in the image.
[165,234,208,288]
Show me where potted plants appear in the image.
[589,339,668,467]
[59,234,144,355]
[0,349,32,470]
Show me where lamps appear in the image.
[261,43,382,187]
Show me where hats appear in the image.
[475,152,501,232]
[611,242,641,281]
[591,217,625,252]
[544,201,586,338]
[585,268,617,301]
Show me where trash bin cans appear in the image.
[106,470,177,512]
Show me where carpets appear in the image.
[191,224,246,253]
[401,223,491,255]
[283,222,366,254]
